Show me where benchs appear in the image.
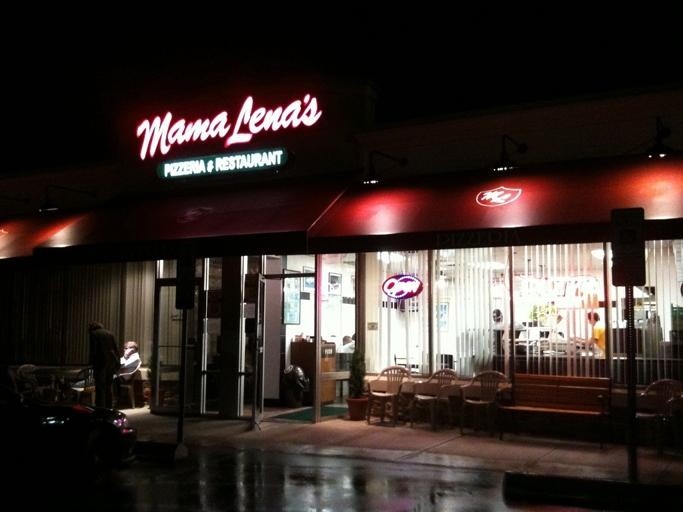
[495,373,613,449]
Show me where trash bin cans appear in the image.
[283,366,306,409]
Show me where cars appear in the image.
[0,383,138,479]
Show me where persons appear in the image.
[87,321,120,409]
[113,340,143,384]
[492,309,506,329]
[570,312,605,355]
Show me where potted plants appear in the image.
[344,347,367,421]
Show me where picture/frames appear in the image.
[281,266,341,326]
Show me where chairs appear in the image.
[366,366,507,438]
[7,360,142,410]
[634,379,682,457]
